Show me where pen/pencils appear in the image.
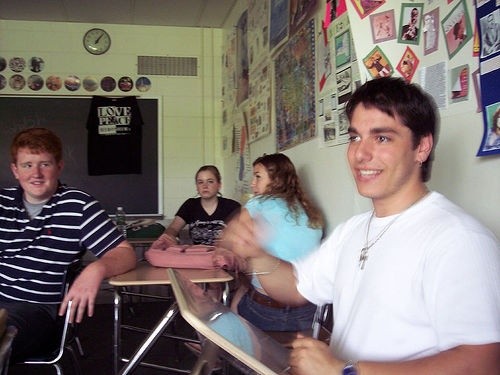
[312,300,326,339]
[283,366,291,373]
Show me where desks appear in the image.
[116,266,291,375]
[125,237,180,261]
[107,261,235,375]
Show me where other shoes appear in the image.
[184,342,202,354]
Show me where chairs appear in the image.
[12,259,87,375]
[0,306,18,375]
[266,303,333,350]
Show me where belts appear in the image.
[248,287,287,309]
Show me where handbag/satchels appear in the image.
[145,235,239,290]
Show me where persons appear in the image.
[217,77,500,375]
[482,15,500,55]
[368,55,388,78]
[486,108,500,150]
[160,153,322,369]
[401,8,421,41]
[0,128,136,375]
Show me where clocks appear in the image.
[83,28,112,56]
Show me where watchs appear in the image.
[342,359,360,375]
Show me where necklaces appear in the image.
[359,188,429,268]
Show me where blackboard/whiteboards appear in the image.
[0,94,163,217]
